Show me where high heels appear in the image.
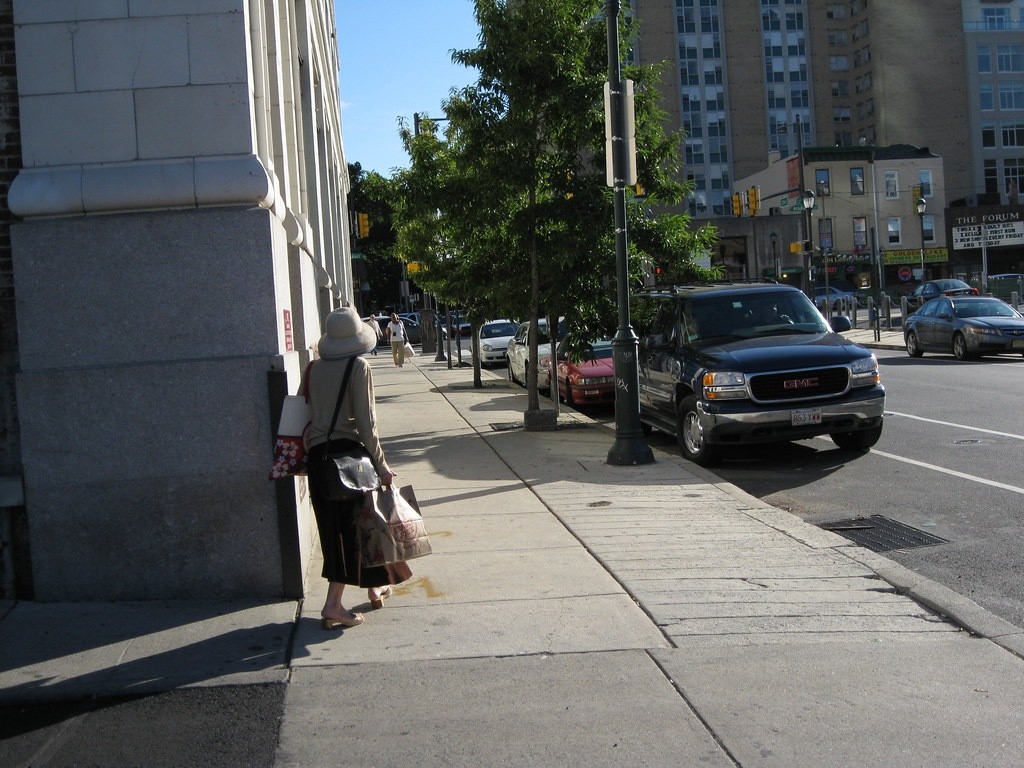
[371,585,392,608]
[321,612,365,629]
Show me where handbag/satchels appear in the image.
[321,454,381,501]
[268,359,315,481]
[404,341,415,358]
[377,480,433,561]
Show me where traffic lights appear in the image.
[746,189,757,210]
[731,194,740,215]
[655,262,671,288]
[355,211,369,238]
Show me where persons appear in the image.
[297,307,415,631]
[385,313,409,367]
[366,314,384,356]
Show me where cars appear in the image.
[906,278,979,313]
[549,331,616,407]
[507,315,589,395]
[359,310,472,345]
[469,319,521,368]
[972,274,1024,304]
[902,295,1024,361]
[802,286,855,312]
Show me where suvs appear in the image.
[630,277,886,467]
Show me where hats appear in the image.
[318,307,377,360]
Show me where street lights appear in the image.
[916,196,927,284]
[769,232,777,279]
[778,114,807,295]
[816,180,829,290]
[801,190,815,307]
[720,244,726,280]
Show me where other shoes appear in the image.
[375,352,378,356]
[399,364,403,367]
[371,352,374,355]
[395,363,399,366]
